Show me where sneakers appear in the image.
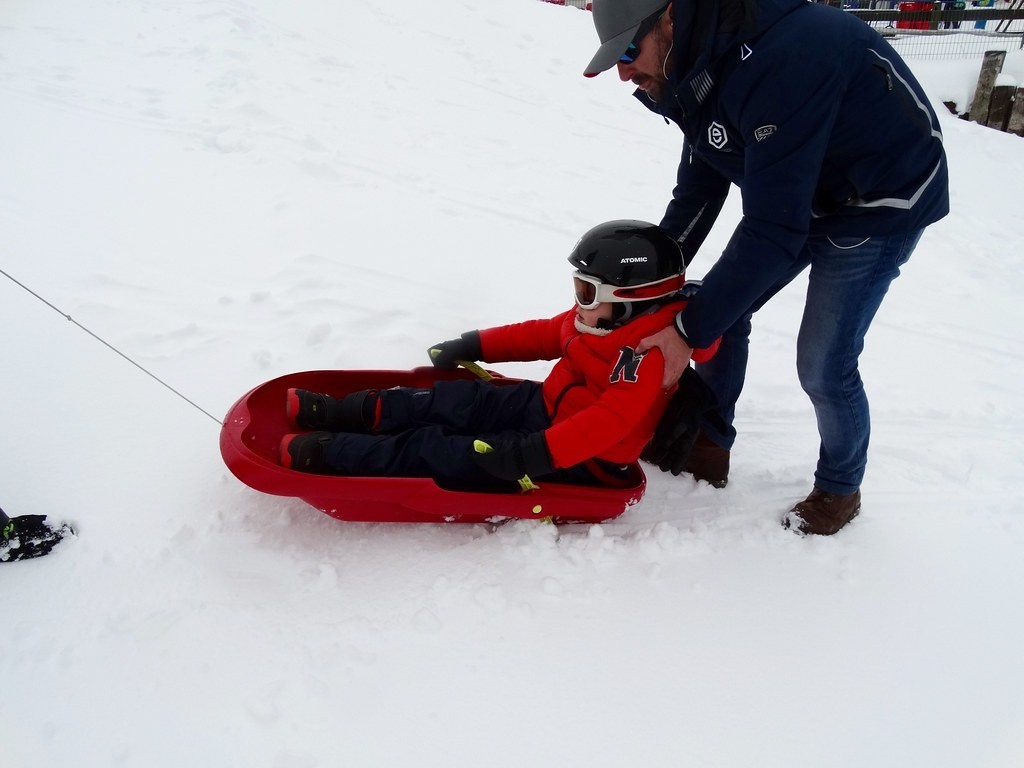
[638,436,731,489]
[0,515,75,563]
[782,486,861,538]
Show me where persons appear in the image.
[583,0,950,536]
[280,220,723,496]
[0,507,75,563]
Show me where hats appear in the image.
[583,0,670,78]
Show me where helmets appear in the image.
[567,219,686,302]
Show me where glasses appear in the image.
[619,25,650,65]
[572,269,602,310]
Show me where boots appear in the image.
[286,387,383,436]
[280,431,336,473]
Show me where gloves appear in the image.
[464,431,549,473]
[426,329,485,370]
